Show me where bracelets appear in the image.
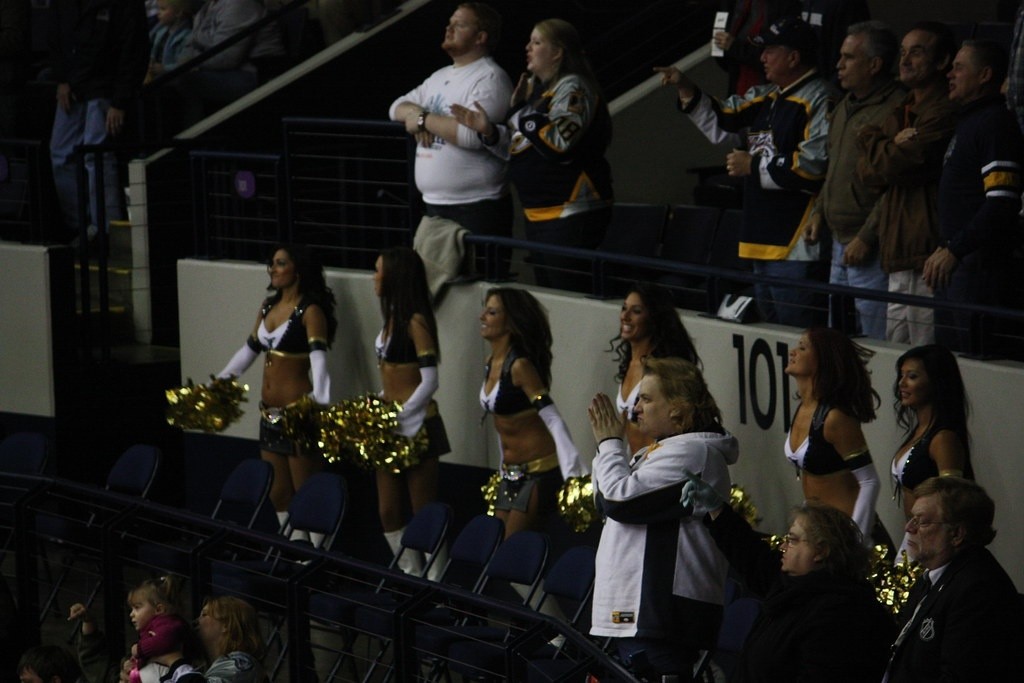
[681,97,692,104]
[418,111,430,129]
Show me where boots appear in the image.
[511,579,567,647]
[383,526,423,580]
[277,511,311,566]
[424,544,447,583]
[308,531,325,548]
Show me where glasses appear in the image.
[906,514,951,528]
[784,534,812,548]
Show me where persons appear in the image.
[370,246,449,606]
[201,242,332,565]
[653,0,1023,360]
[887,475,1024,683]
[0,570,270,683]
[449,19,615,292]
[679,468,898,683]
[388,0,517,282]
[886,344,996,570]
[479,286,589,646]
[588,358,739,683]
[607,283,698,463]
[784,325,898,563]
[0,1,385,252]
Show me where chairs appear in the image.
[0,0,1024,683]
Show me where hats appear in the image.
[747,14,824,60]
